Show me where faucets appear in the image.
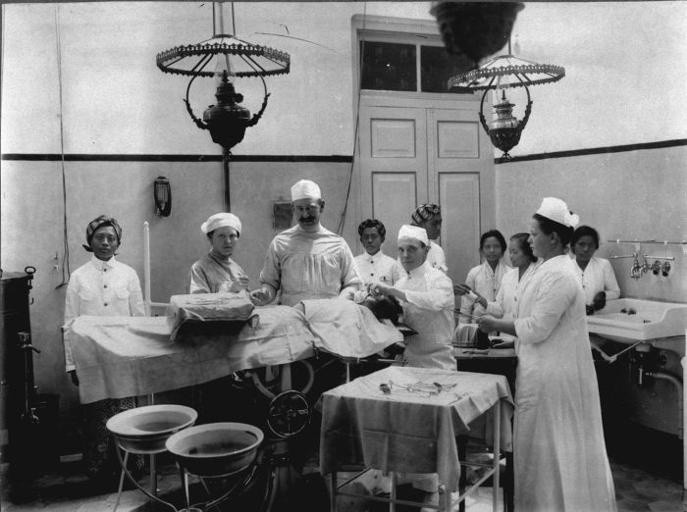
[608,253,639,278]
[639,254,675,278]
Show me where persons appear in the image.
[458,229,512,323]
[452,232,532,326]
[473,196,621,511]
[365,223,458,373]
[61,215,148,473]
[346,219,400,294]
[65,293,400,355]
[566,225,621,316]
[187,211,248,301]
[245,180,363,310]
[394,202,449,275]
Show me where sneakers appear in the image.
[143,459,179,477]
[421,492,440,512]
[132,472,173,494]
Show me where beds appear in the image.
[61,298,405,510]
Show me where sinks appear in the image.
[585,295,687,346]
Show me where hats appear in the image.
[290,179,322,202]
[411,202,441,239]
[536,196,579,228]
[397,224,428,246]
[206,212,242,239]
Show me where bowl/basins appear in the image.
[107,405,197,451]
[164,419,265,479]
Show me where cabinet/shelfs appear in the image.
[0,265,41,490]
[176,457,264,511]
[109,436,192,507]
[315,366,515,511]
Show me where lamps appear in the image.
[447,37,566,164]
[156,2,291,157]
